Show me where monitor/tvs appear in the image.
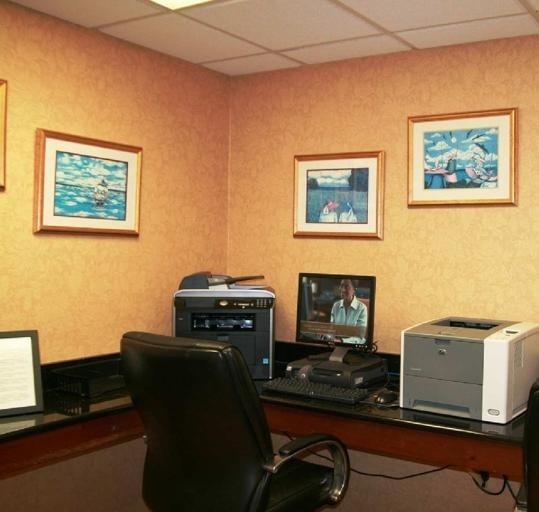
[295,273,376,363]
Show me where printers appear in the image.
[172,271,276,381]
[399,316,539,425]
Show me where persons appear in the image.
[330,278,366,344]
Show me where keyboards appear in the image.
[261,377,370,404]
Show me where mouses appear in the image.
[375,390,398,404]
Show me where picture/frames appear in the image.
[405,107,522,209]
[32,127,144,237]
[292,149,387,240]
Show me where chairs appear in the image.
[120,331,352,512]
